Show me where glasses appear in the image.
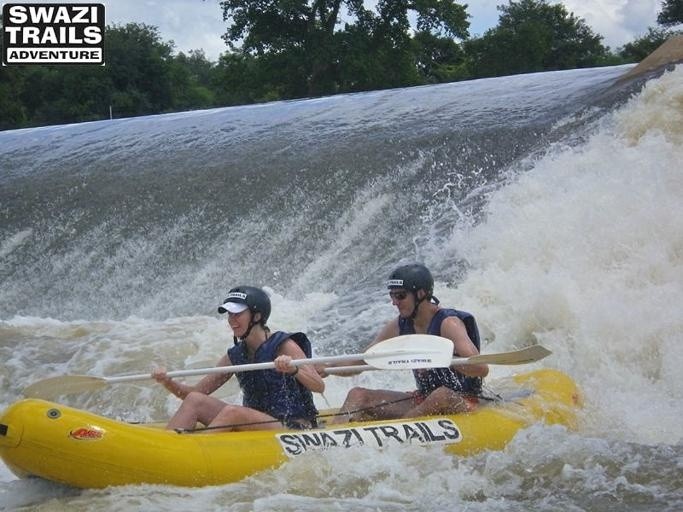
[391,291,409,301]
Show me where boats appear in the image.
[2,361,588,499]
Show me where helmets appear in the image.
[388,265,435,296]
[217,285,271,324]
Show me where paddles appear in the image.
[326,344,552,374]
[21,333,454,399]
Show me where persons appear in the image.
[147,285,327,439]
[312,261,490,427]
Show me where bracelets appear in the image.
[287,366,299,377]
